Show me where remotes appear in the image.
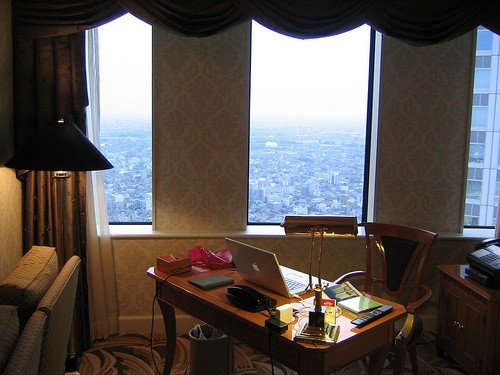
[351,304,393,326]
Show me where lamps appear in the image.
[5,113,114,182]
[280,214,359,290]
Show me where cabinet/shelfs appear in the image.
[436,264,500,375]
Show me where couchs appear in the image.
[2,254,82,375]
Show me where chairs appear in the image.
[363,221,438,375]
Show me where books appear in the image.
[187,274,235,290]
[324,280,364,302]
[337,296,382,314]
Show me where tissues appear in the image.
[155,253,192,276]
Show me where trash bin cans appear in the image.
[189,325,232,375]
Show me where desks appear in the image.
[146,264,407,375]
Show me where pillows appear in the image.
[0,244,59,335]
[0,304,19,374]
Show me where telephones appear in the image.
[226,284,277,313]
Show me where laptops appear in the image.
[225,237,325,298]
